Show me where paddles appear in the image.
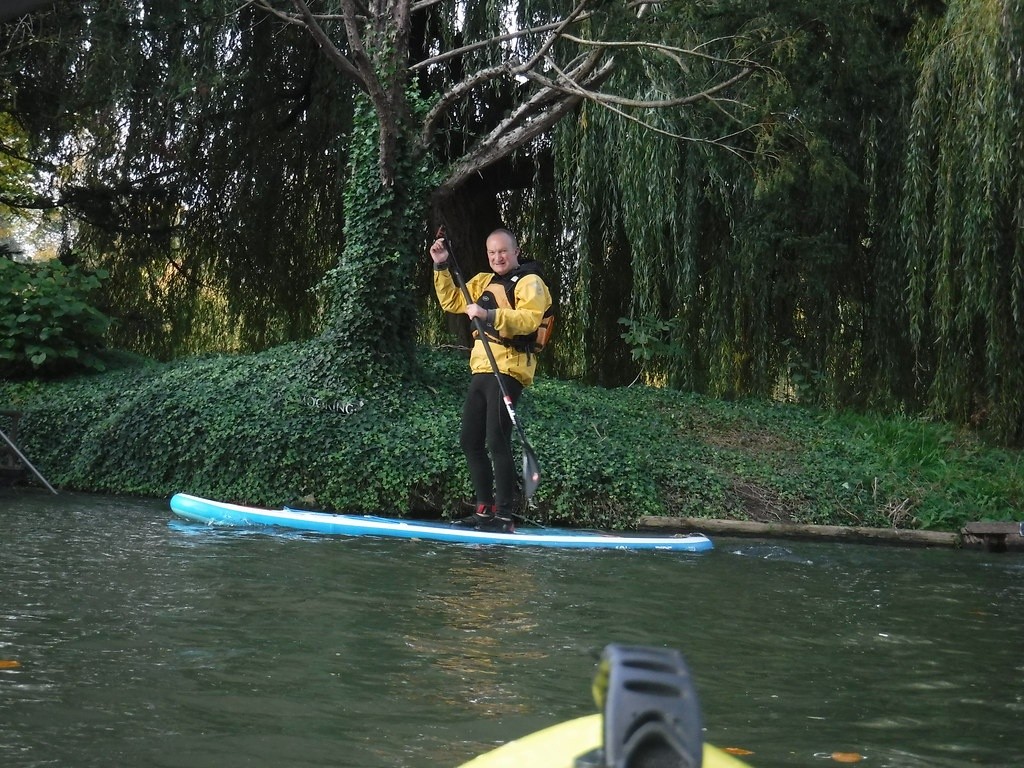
[436,225,542,498]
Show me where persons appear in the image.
[430,229,552,534]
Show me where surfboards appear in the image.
[170,493,713,552]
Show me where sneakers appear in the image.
[453,512,515,533]
